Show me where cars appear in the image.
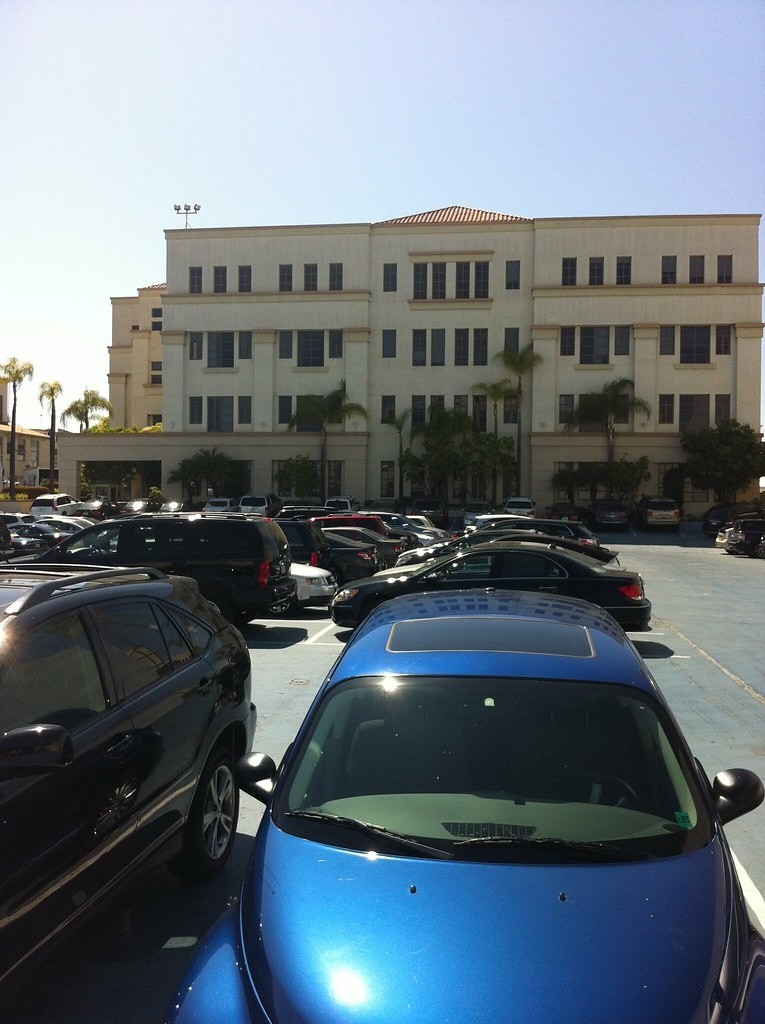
[165,586,765,1024]
[701,501,765,559]
[0,493,622,610]
[326,539,653,632]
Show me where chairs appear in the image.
[512,689,628,807]
[345,688,467,798]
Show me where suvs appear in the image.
[0,510,299,626]
[586,497,630,533]
[632,495,682,533]
[0,555,258,1002]
